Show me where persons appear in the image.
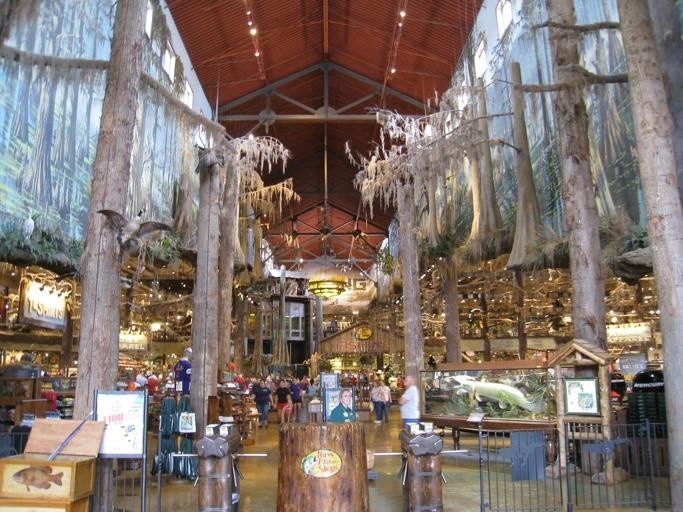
[173,357,191,394]
[364,370,404,424]
[398,376,421,426]
[123,370,159,395]
[235,374,319,428]
[329,390,353,422]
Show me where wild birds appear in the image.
[23,208,35,242]
[96,206,173,245]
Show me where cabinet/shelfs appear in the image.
[1,374,76,428]
[208,380,262,447]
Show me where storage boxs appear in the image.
[0,496,90,512]
[0,416,106,503]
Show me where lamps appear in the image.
[30,277,73,299]
[304,121,352,300]
[119,324,142,336]
